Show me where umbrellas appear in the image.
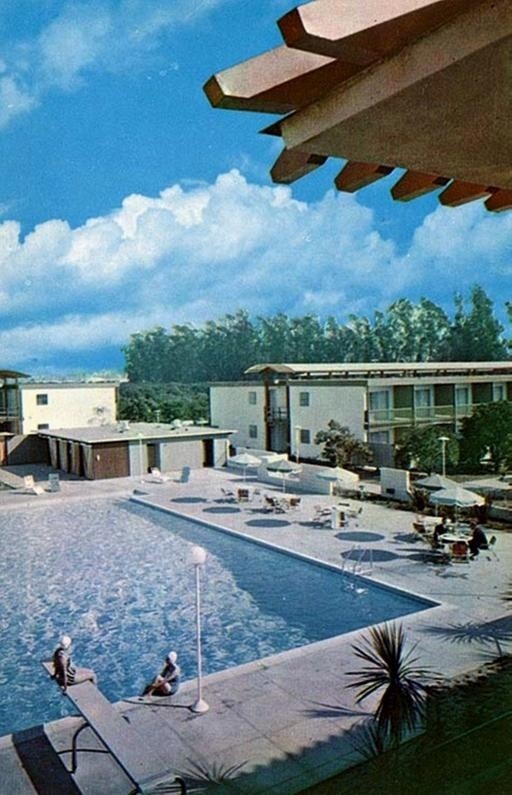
[410,474,462,517]
[429,486,486,525]
[266,458,302,493]
[227,451,262,483]
[316,467,359,497]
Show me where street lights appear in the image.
[189,544,209,712]
[438,436,450,477]
[294,425,302,462]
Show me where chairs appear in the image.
[313,503,363,530]
[141,466,190,484]
[410,520,500,568]
[221,488,301,515]
[48,473,61,493]
[23,474,43,495]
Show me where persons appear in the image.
[143,651,181,699]
[467,521,488,556]
[433,516,449,549]
[50,635,97,694]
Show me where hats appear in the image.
[167,651,177,665]
[60,636,72,650]
